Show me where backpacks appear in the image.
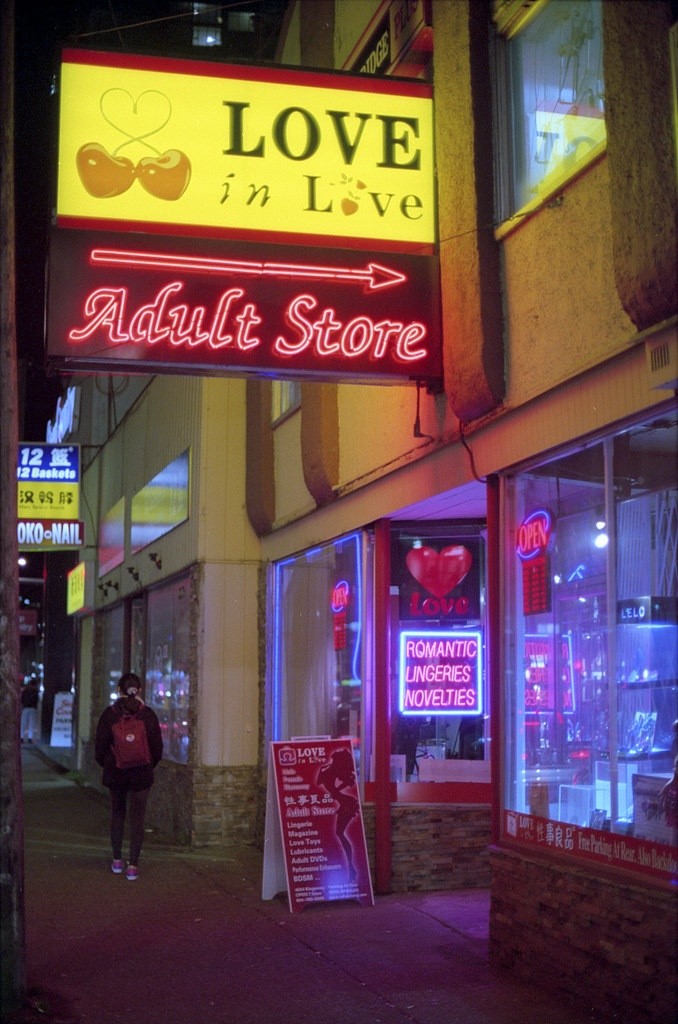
[105,703,151,770]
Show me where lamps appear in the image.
[126,567,139,580]
[98,583,107,594]
[104,580,117,589]
[148,552,162,568]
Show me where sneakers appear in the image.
[112,859,124,873]
[125,865,138,881]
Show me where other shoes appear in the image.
[28,739,32,744]
[21,738,24,744]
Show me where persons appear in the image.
[94,673,164,880]
[20,680,38,744]
